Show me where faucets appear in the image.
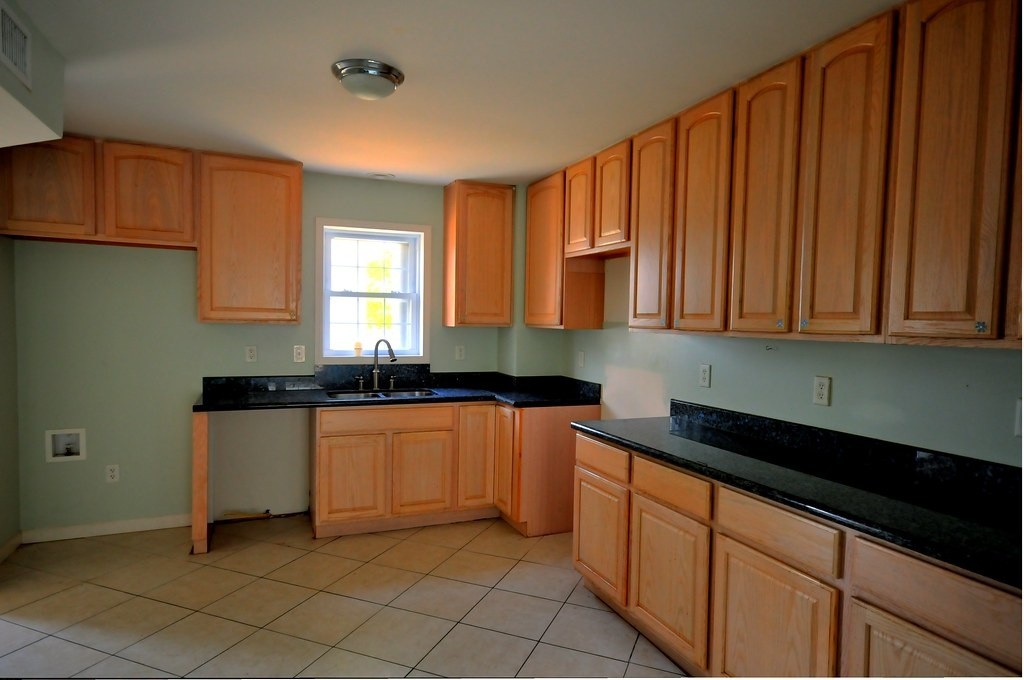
[372,339,398,390]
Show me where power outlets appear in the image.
[814,377,831,406]
[294,345,305,362]
[106,464,119,483]
[698,364,711,388]
[245,346,258,361]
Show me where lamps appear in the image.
[331,57,405,100]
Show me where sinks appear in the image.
[380,391,438,398]
[327,393,385,399]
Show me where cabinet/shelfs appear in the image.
[443,179,516,326]
[0,136,302,324]
[525,1,1022,349]
[308,401,1022,677]
